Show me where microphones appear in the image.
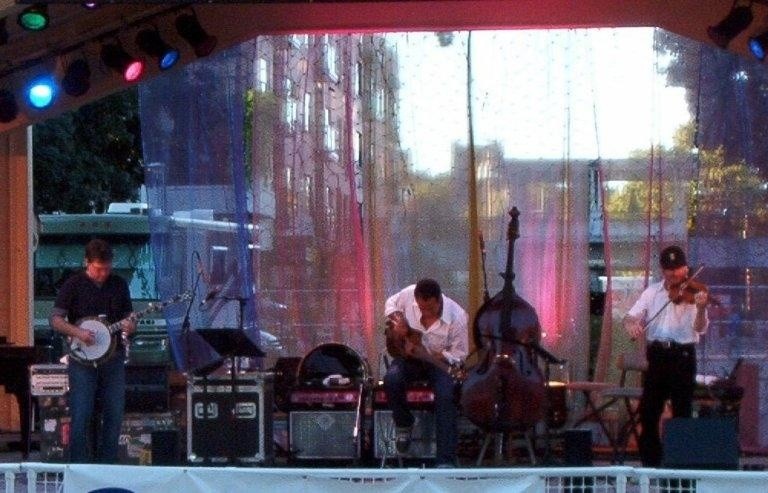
[199,284,223,307]
[196,252,211,283]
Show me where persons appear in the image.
[384,279,469,468]
[726,304,741,356]
[622,246,710,467]
[49,239,137,463]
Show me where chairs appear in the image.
[606,352,652,464]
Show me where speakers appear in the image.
[660,416,740,469]
[374,411,439,459]
[289,411,361,459]
[565,428,593,465]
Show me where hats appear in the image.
[659,245,686,269]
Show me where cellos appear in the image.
[460,207,547,434]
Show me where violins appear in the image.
[668,277,721,307]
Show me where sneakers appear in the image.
[395,423,413,453]
[437,463,455,469]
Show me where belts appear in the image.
[648,341,695,350]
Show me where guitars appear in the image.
[386,311,467,385]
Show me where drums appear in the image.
[273,357,305,413]
[296,342,373,412]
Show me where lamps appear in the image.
[18,2,218,108]
